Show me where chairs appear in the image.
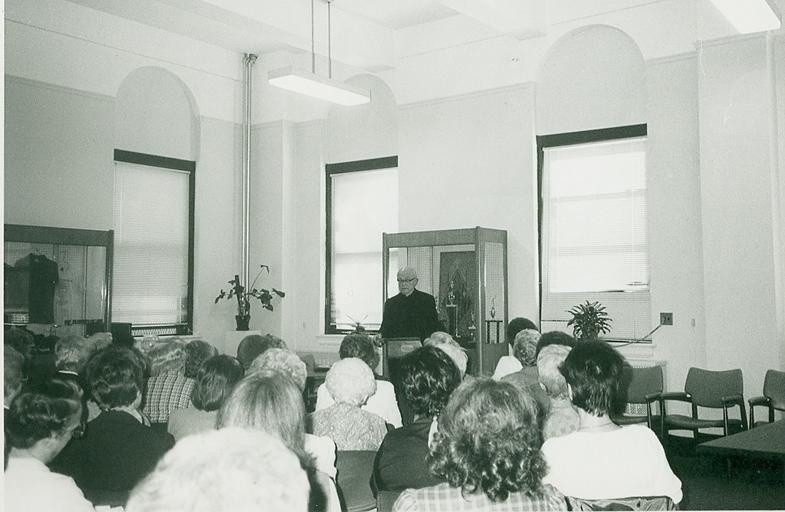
[619,364,670,440]
[656,363,751,456]
[335,451,400,511]
[745,369,785,432]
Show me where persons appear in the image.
[373,266,446,341]
[3,314,684,512]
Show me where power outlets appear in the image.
[659,312,674,326]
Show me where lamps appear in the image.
[266,0,373,108]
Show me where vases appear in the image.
[234,313,252,332]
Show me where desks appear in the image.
[697,420,781,466]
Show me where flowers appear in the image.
[213,263,286,317]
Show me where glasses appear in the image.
[397,278,415,284]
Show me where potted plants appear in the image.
[566,300,613,340]
